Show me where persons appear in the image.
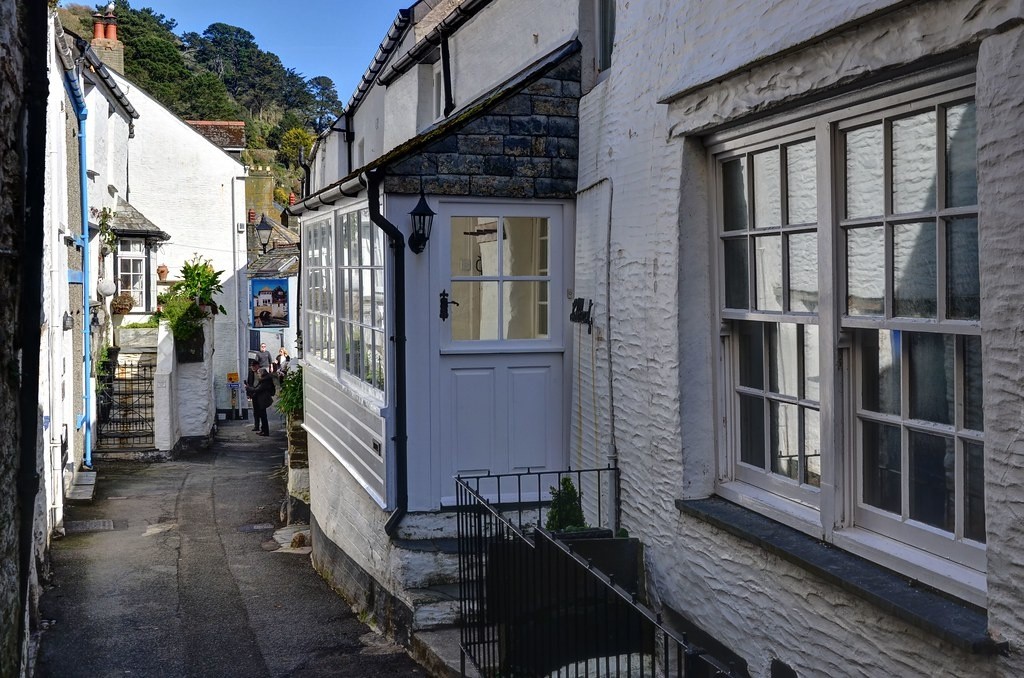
[243,368,276,436]
[274,347,291,391]
[256,342,274,373]
[246,359,263,432]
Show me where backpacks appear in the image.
[259,379,276,396]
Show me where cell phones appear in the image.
[244,380,247,386]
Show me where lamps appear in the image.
[408,194,434,255]
[569,298,594,333]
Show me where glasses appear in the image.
[262,346,266,348]
[279,351,283,353]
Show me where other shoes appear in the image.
[280,387,284,392]
[260,432,269,436]
[256,432,263,434]
[252,428,260,431]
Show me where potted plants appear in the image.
[164,261,228,364]
[487,477,612,573]
[97,346,120,422]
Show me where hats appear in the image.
[251,361,259,366]
